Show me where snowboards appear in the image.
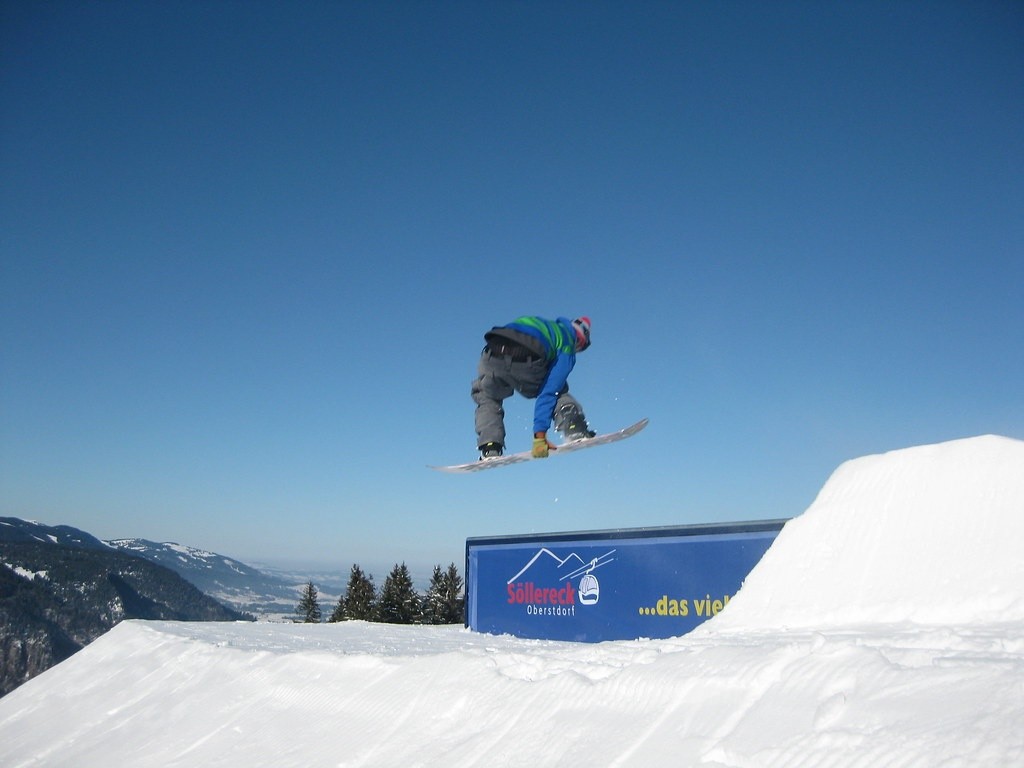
[426,417,650,475]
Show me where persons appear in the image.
[471,316,597,461]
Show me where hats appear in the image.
[571,316,591,351]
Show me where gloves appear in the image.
[533,436,557,457]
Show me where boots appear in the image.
[561,403,597,440]
[478,442,501,460]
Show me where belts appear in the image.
[482,345,542,364]
